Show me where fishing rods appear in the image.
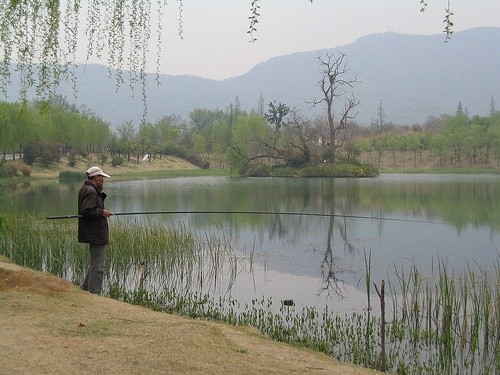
[45,211,455,226]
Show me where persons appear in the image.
[77,166,114,295]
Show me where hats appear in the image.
[85,166,111,179]
